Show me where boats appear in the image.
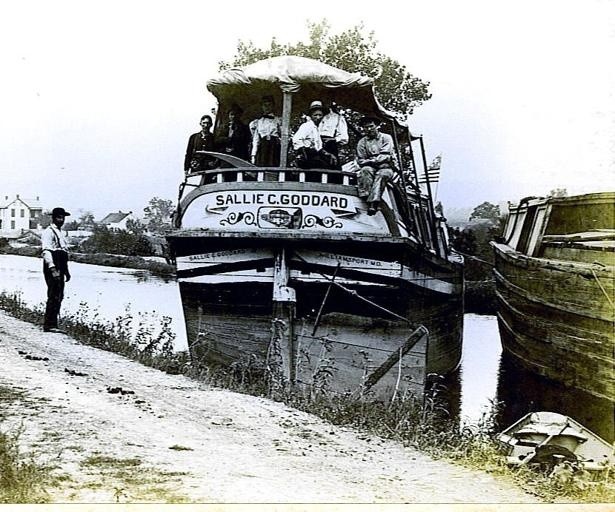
[161,54,469,408]
[491,191,615,411]
[485,408,614,489]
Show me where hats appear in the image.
[306,101,329,115]
[360,114,380,120]
[260,96,275,102]
[49,208,70,216]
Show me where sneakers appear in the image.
[366,199,381,216]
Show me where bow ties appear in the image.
[263,114,274,119]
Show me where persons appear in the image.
[41,207,71,331]
[184,89,399,215]
